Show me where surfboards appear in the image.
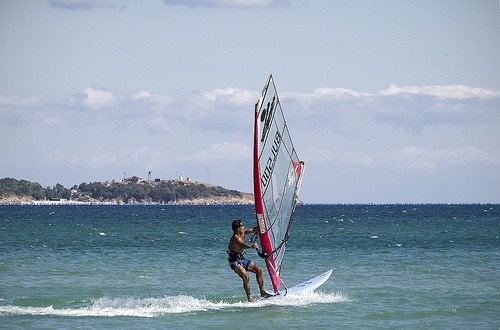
[257,269,333,303]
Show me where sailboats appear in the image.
[245,73,332,303]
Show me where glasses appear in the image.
[239,224,244,227]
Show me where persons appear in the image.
[226,220,274,303]
